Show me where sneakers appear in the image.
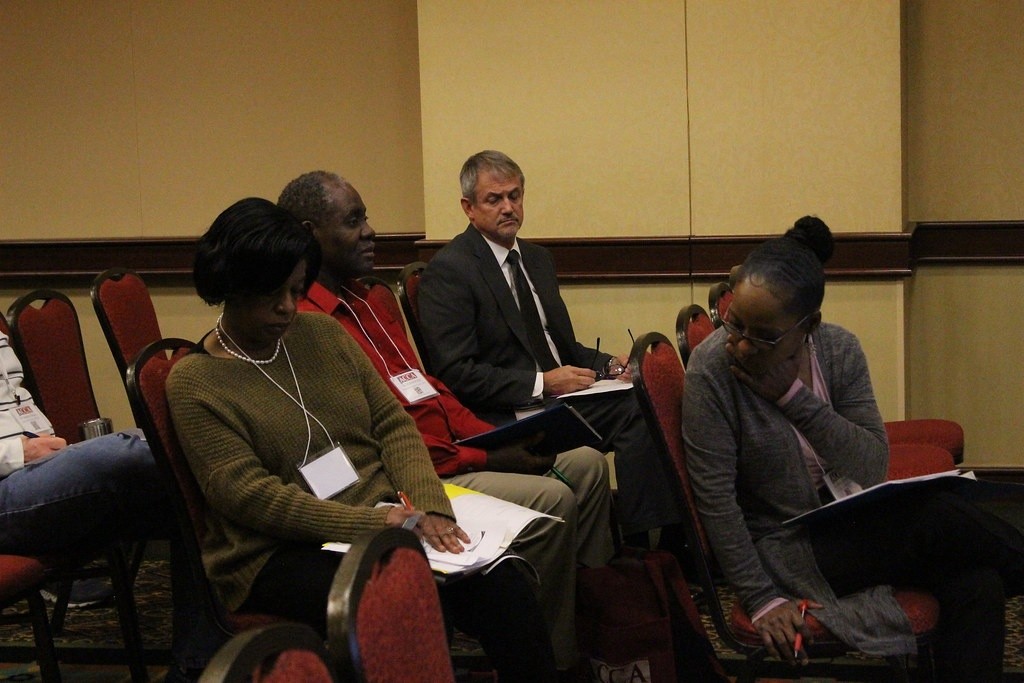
[37,570,116,609]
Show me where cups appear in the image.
[77,418,114,442]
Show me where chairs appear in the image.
[628,283,968,683]
[0,267,466,683]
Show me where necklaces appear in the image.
[214,312,280,365]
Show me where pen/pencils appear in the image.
[604,376,617,380]
[23,431,40,438]
[531,447,577,492]
[397,491,411,510]
[794,598,808,659]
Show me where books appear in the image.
[451,403,604,456]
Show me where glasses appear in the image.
[722,301,818,351]
[591,328,635,382]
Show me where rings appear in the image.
[447,526,454,532]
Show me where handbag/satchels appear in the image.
[575,545,731,683]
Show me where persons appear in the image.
[417,152,681,553]
[0,329,229,683]
[165,171,616,683]
[682,215,1024,683]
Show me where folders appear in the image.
[450,402,604,444]
[781,469,1024,528]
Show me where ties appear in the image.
[506,249,560,372]
